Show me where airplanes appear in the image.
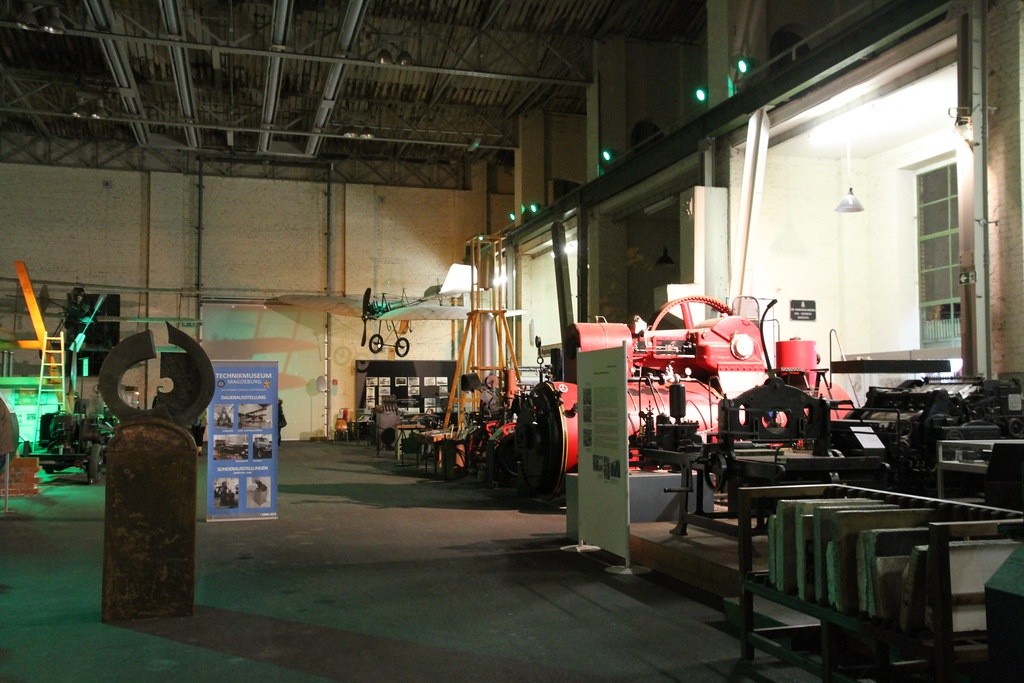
[276,263,524,358]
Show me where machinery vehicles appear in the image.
[28,392,119,486]
